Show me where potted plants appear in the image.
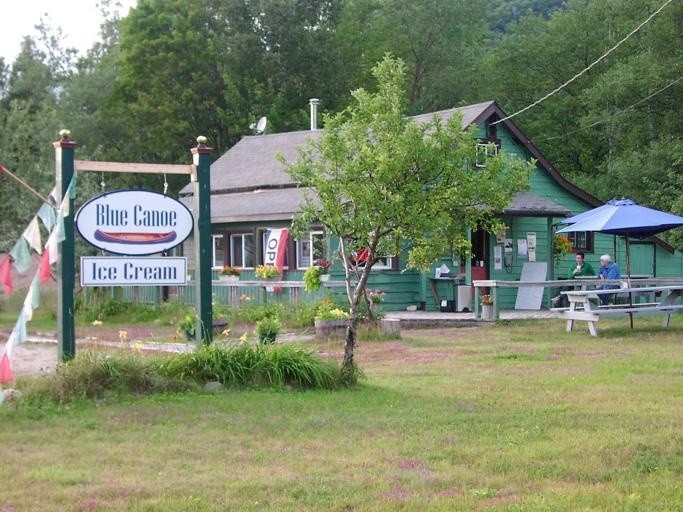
[254,316,281,346]
[254,263,281,282]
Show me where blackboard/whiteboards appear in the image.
[514,262,547,310]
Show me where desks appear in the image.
[427,276,464,310]
[559,285,683,337]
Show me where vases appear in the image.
[219,274,240,282]
[318,273,329,282]
[480,304,493,321]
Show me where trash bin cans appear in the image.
[455,284,472,312]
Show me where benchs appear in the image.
[547,301,681,317]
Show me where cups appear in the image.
[476,260,484,268]
[576,265,581,273]
[620,280,628,289]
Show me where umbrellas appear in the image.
[556,196,621,264]
[555,196,683,287]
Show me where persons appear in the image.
[549,251,595,306]
[594,253,622,309]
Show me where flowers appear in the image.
[479,294,493,305]
[303,258,332,294]
[553,231,574,270]
[364,287,386,311]
[217,264,240,275]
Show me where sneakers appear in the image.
[550,295,561,306]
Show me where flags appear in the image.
[0,167,78,413]
